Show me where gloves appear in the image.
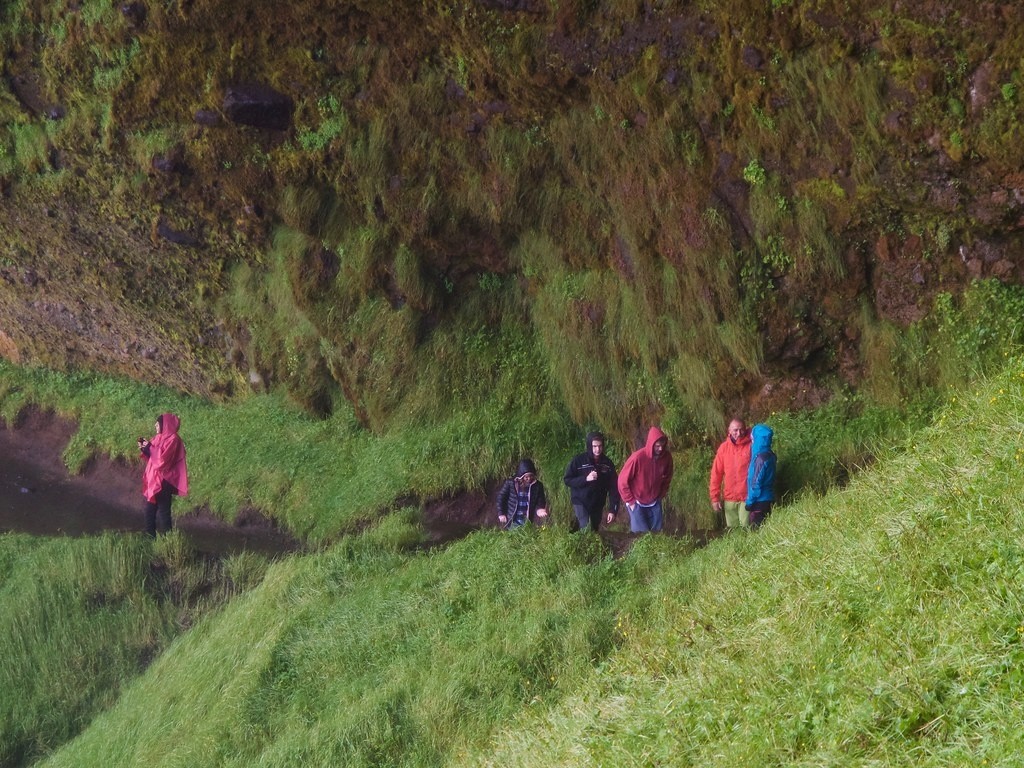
[745,505,752,511]
[712,501,722,513]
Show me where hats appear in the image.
[157,415,163,423]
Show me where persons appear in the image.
[137,412,189,538]
[563,430,621,529]
[617,426,673,533]
[709,418,778,528]
[496,458,548,531]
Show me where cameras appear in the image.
[137,437,144,446]
